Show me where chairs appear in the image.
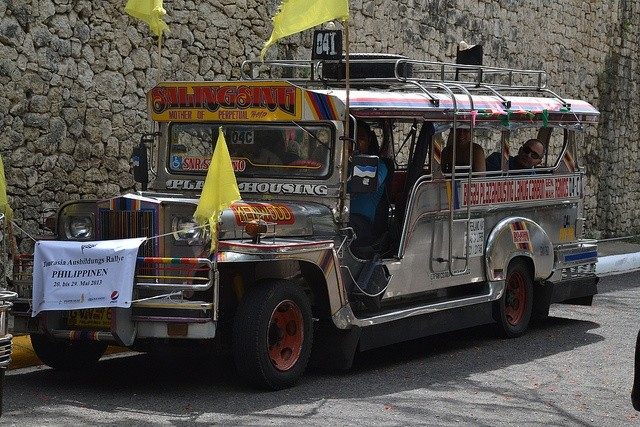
[352,157,395,260]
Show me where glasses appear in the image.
[521,146,541,159]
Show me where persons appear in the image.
[485,139,546,171]
[347,122,389,242]
[440,124,486,174]
